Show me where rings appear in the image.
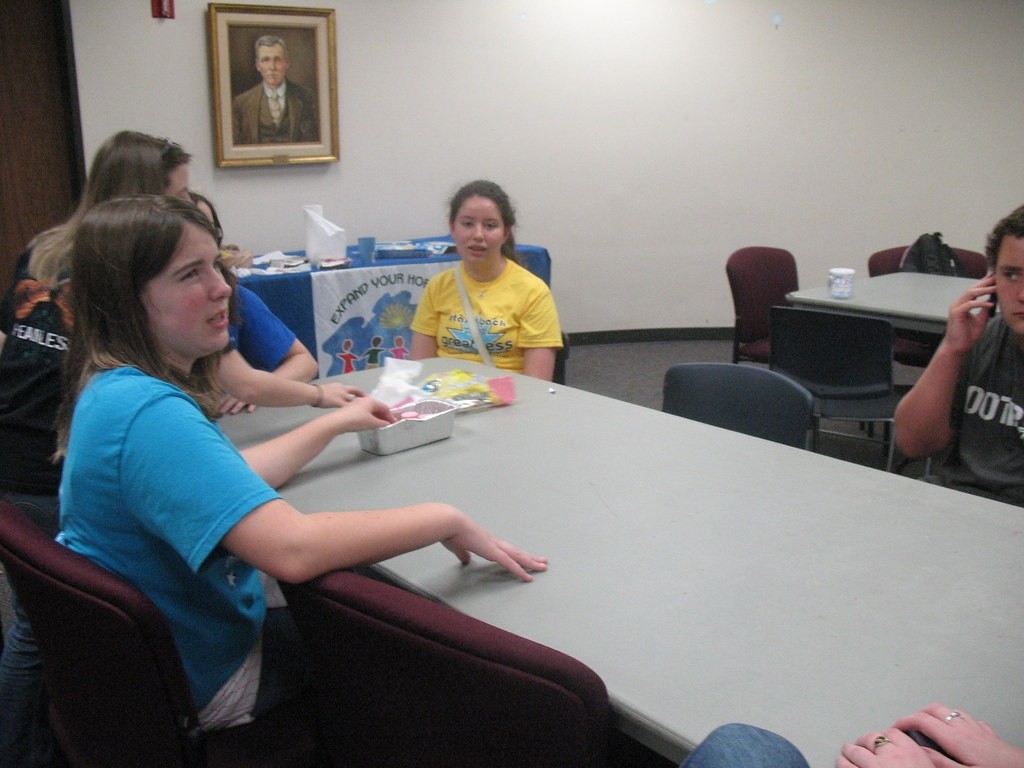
[874,736,891,753]
[945,712,961,723]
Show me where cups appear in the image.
[357,237,376,267]
[827,267,856,300]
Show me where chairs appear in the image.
[867,245,987,369]
[767,305,933,477]
[661,361,815,451]
[0,499,316,768]
[276,567,611,768]
[726,246,800,373]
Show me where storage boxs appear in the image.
[358,399,460,456]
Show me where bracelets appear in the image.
[308,384,323,409]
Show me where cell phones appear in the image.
[987,292,998,318]
[285,258,307,267]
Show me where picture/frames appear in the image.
[208,2,340,168]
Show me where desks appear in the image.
[221,358,1024,768]
[228,237,550,372]
[784,270,1000,334]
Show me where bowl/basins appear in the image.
[356,400,458,454]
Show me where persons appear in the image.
[895,205,1024,509]
[0,129,364,768]
[54,194,549,768]
[184,194,319,415]
[678,701,1023,768]
[410,180,564,381]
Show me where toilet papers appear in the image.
[302,215,350,268]
